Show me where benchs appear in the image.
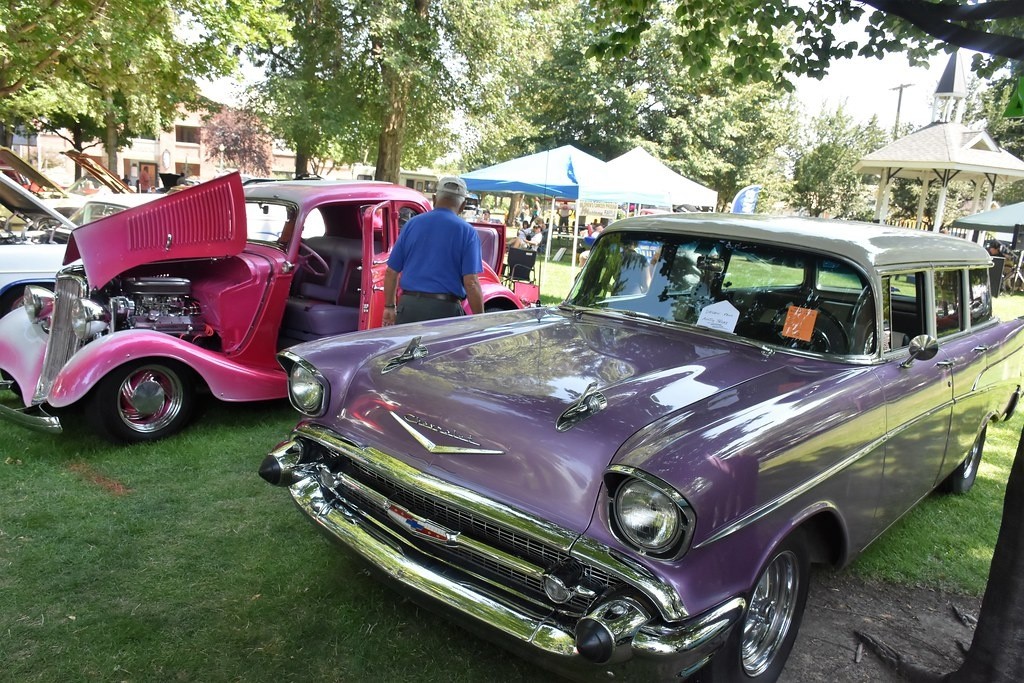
[280,238,383,342]
[555,226,589,236]
[743,290,918,340]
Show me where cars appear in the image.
[259,210,1024,683]
[0,143,531,447]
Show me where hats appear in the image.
[436,176,467,196]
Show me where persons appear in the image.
[382,176,485,326]
[119,166,188,193]
[941,228,1005,258]
[482,197,698,297]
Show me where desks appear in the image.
[558,234,585,247]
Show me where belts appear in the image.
[401,290,462,305]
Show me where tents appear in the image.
[953,202,1024,245]
[570,146,717,271]
[462,145,607,262]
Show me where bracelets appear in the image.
[384,304,396,309]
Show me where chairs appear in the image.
[842,321,910,355]
[500,248,537,289]
